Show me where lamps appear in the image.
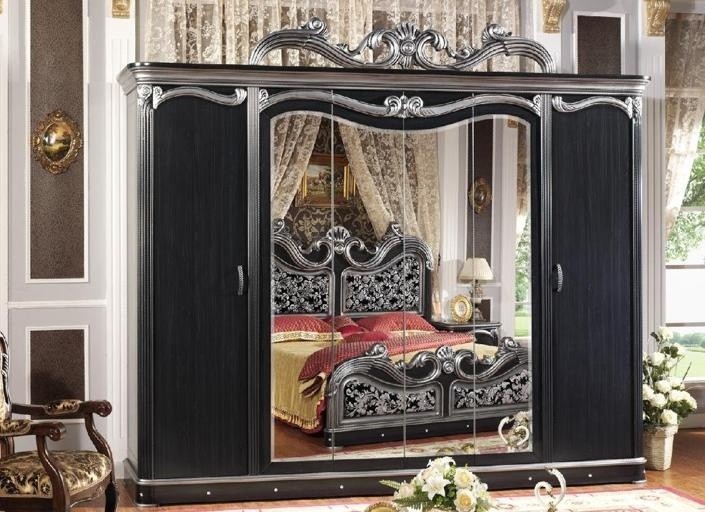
[458,257,494,321]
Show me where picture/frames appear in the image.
[293,152,356,209]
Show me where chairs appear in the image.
[1,330,117,512]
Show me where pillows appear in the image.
[360,312,440,335]
[322,312,363,336]
[272,314,343,343]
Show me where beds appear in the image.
[271,218,534,448]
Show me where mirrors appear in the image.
[260,89,537,472]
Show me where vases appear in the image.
[643,425,680,471]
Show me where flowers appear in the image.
[378,451,503,512]
[642,321,699,434]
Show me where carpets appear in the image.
[199,484,703,512]
[275,434,511,462]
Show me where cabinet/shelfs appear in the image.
[545,95,641,463]
[124,84,252,478]
